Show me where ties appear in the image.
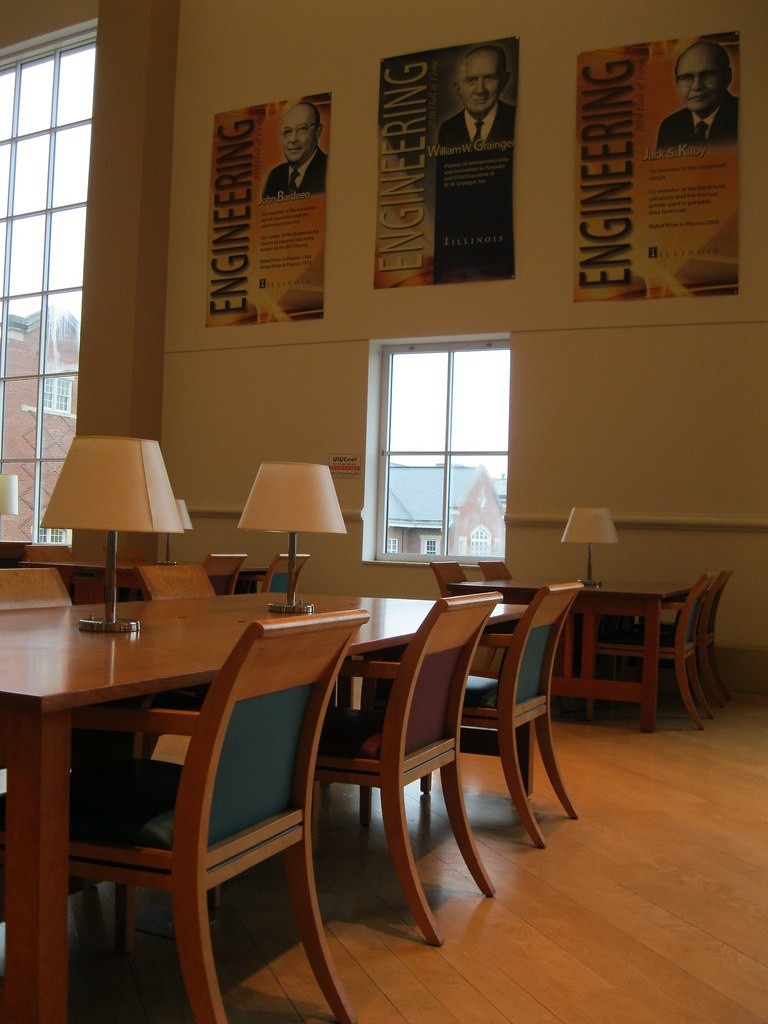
[696,120,708,145]
[472,121,484,146]
[288,170,300,195]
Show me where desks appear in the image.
[20,559,268,604]
[447,576,695,733]
[0,590,530,1024]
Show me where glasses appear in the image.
[279,123,315,139]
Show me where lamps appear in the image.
[38,435,186,633]
[152,498,194,565]
[561,506,619,586]
[0,474,18,537]
[237,461,348,613]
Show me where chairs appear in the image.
[0,551,735,1024]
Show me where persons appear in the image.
[657,42,738,150]
[263,102,327,198]
[436,45,515,144]
